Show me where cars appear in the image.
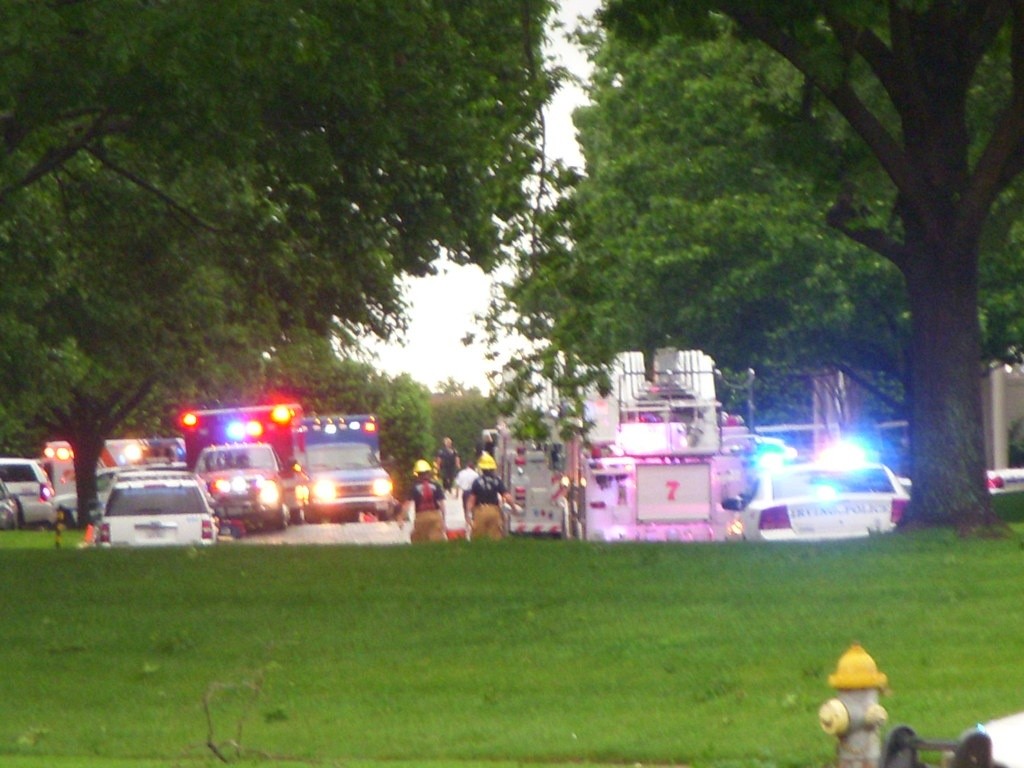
[0,477,19,529]
[95,470,220,548]
[722,450,910,541]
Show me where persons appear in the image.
[397,458,445,544]
[436,437,461,493]
[455,464,479,514]
[466,454,519,541]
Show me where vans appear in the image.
[40,437,186,496]
[0,458,55,529]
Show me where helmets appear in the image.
[412,460,431,473]
[477,455,497,470]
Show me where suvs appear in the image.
[304,442,393,524]
[193,442,290,530]
[54,457,207,530]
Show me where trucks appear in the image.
[184,403,380,526]
[483,348,749,543]
